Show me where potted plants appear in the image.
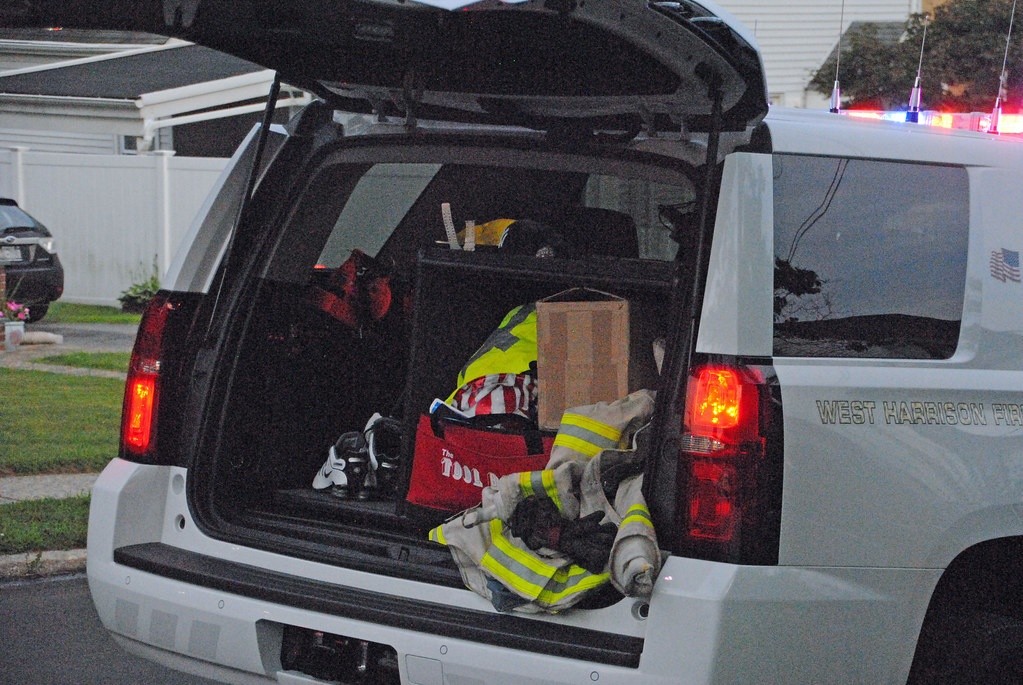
[118,254,160,314]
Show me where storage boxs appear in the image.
[534,282,633,431]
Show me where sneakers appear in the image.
[312,413,401,497]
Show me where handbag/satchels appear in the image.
[404,404,558,515]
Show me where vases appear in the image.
[3,320,26,352]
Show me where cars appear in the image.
[0,196,63,323]
[45,4,1021,685]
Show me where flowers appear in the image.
[0,297,31,322]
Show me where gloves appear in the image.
[512,496,615,576]
